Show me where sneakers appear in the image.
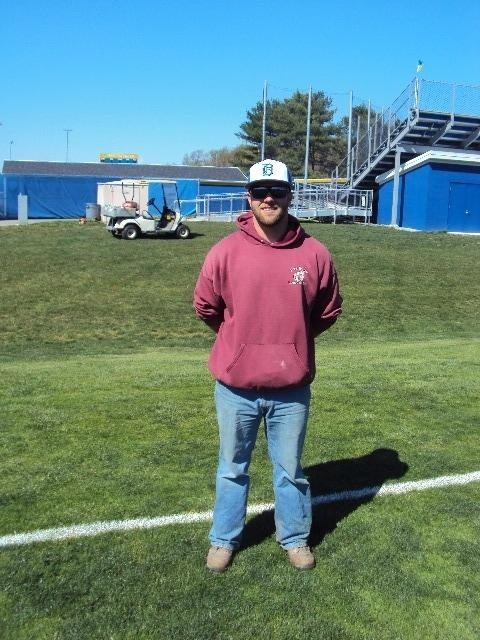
[206,544,233,573]
[286,544,316,571]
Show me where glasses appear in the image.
[250,186,290,199]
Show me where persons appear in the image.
[192,156,346,573]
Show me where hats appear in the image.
[244,158,292,190]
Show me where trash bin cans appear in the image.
[85,203,101,222]
